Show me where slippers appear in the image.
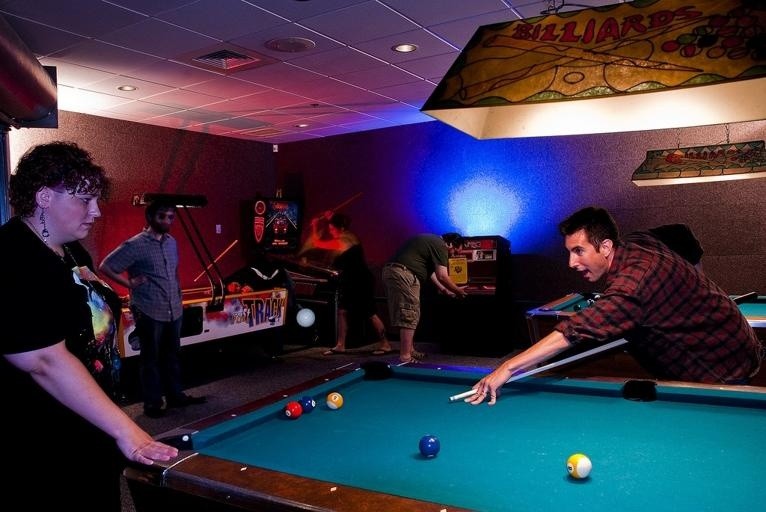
[322,347,346,355]
[372,347,399,355]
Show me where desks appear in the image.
[123,359,766,512]
[526,291,766,387]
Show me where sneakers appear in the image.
[144,394,207,419]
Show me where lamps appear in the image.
[419,0,766,141]
[631,121,766,188]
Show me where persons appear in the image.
[381,232,471,364]
[0,139,180,510]
[205,244,302,355]
[97,199,207,418]
[308,209,393,356]
[463,204,766,406]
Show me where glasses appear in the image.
[156,210,174,220]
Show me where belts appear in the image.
[385,262,405,269]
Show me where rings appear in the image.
[481,389,489,395]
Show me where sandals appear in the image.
[401,349,430,364]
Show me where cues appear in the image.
[449,291,757,402]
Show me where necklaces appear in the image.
[22,214,108,304]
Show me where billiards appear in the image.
[420,435,440,459]
[567,453,593,479]
[284,400,304,418]
[327,393,343,409]
[301,396,316,413]
